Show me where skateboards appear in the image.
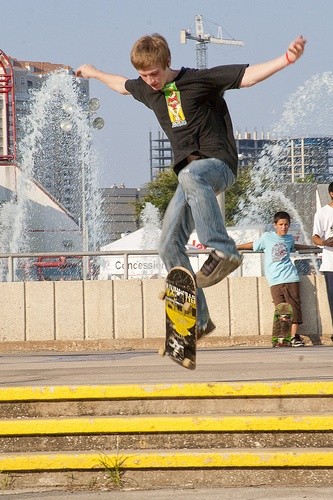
[272,303,292,347]
[160,268,198,369]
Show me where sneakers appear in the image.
[291,336,305,348]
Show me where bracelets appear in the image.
[285,51,294,64]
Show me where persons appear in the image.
[73,33,308,342]
[312,182,332,326]
[235,211,319,347]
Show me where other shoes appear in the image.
[194,250,243,288]
[196,321,216,341]
